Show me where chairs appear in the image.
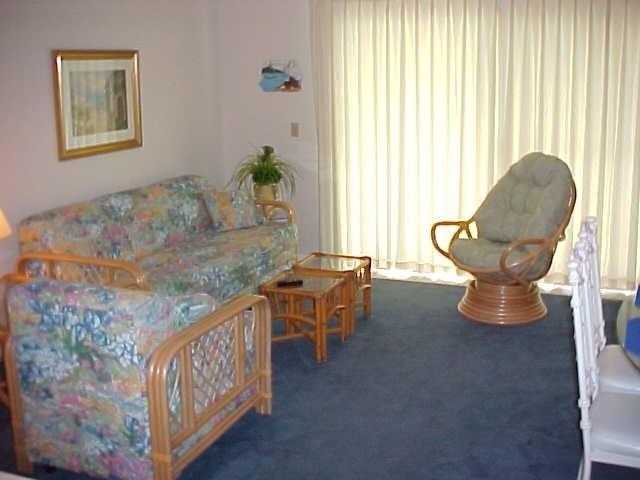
[567,240,639,480]
[430,151,577,327]
[577,216,640,392]
[3,276,274,480]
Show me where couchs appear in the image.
[17,174,301,308]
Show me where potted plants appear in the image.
[222,144,305,201]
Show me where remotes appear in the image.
[278,280,303,286]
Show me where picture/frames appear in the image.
[52,47,144,162]
